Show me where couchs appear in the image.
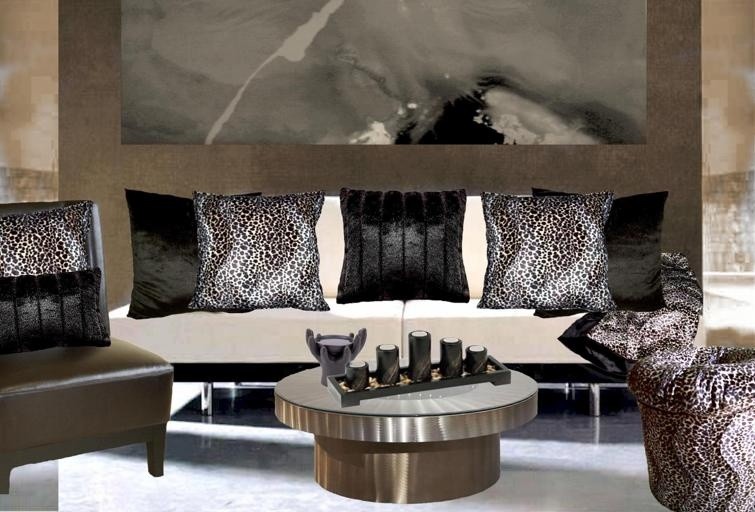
[105,249,695,419]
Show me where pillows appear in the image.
[336,187,470,305]
[188,189,333,313]
[123,184,265,320]
[0,201,94,277]
[531,185,669,311]
[477,189,620,310]
[0,267,110,353]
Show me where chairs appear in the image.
[0,197,175,495]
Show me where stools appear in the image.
[626,346,755,512]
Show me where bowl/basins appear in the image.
[317,335,353,356]
